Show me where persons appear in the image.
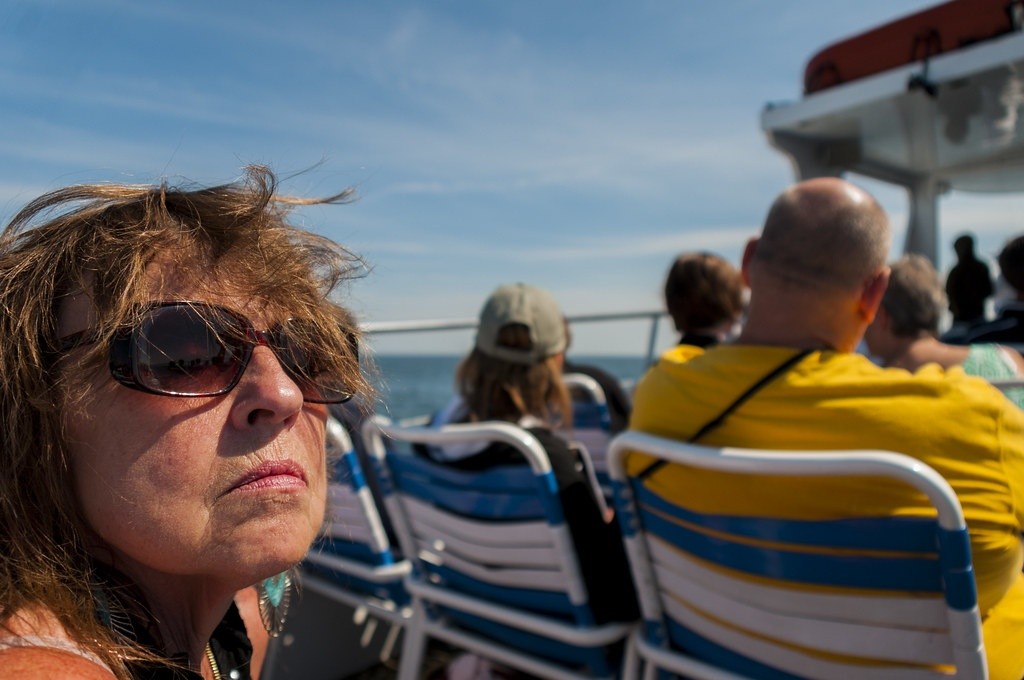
[426,282,620,680]
[664,231,1024,400]
[0,165,357,680]
[633,182,1023,680]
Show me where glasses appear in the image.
[46,300,359,406]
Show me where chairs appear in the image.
[290,371,992,680]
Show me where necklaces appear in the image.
[49,570,221,680]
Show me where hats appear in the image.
[477,283,568,360]
